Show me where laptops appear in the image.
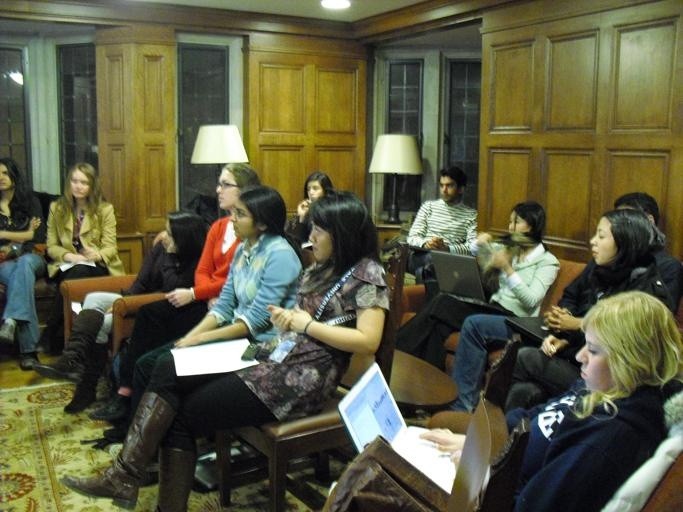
[430,251,504,312]
[504,316,555,342]
[337,362,462,496]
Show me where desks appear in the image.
[333,344,460,414]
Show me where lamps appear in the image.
[187,123,250,180]
[319,0,351,12]
[366,132,424,227]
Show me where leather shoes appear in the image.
[0,322,15,344]
[17,353,40,371]
[87,394,133,421]
[102,426,128,443]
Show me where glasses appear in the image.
[214,182,241,192]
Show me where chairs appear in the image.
[201,239,407,512]
[60,274,169,355]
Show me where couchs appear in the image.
[406,256,589,385]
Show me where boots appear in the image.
[63,342,108,413]
[31,309,104,385]
[60,391,176,510]
[138,446,197,512]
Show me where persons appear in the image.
[395,199,563,374]
[450,190,682,419]
[405,165,479,283]
[503,208,677,414]
[47,162,126,357]
[0,157,45,372]
[34,162,392,511]
[420,289,682,512]
